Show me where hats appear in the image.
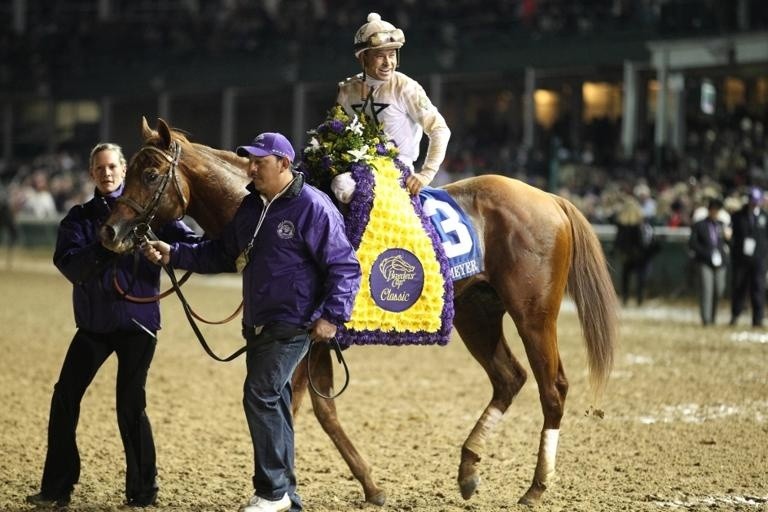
[236,132,296,163]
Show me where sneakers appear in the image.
[27,492,69,508]
[240,493,294,512]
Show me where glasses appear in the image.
[369,29,406,47]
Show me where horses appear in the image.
[98,115,623,508]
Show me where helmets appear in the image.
[353,11,405,57]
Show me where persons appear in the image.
[141,131,363,512]
[1,142,97,254]
[329,10,453,196]
[416,98,768,329]
[26,143,209,507]
